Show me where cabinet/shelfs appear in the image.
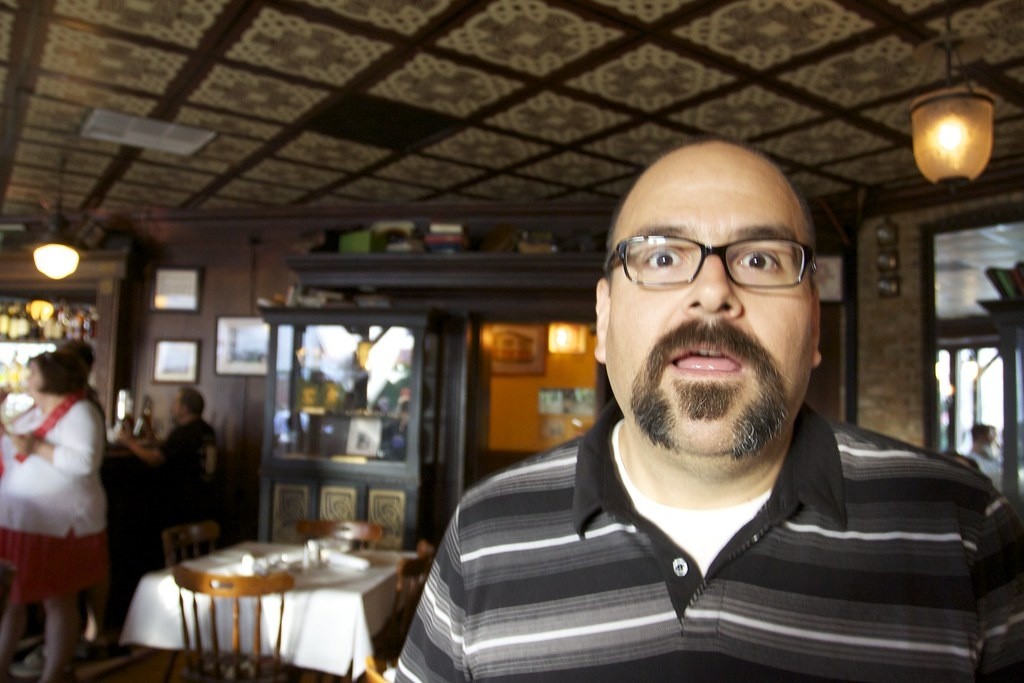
[255,303,448,554]
[280,253,614,490]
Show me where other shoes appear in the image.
[75,634,108,663]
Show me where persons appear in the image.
[966,422,1001,491]
[122,386,217,531]
[0,336,118,683]
[391,139,1024,683]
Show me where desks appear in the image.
[118,543,418,683]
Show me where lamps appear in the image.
[906,13,998,204]
[32,155,80,281]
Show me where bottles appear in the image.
[0,300,97,341]
[133,398,152,438]
[0,349,33,392]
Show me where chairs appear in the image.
[161,435,435,683]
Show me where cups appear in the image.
[307,540,320,567]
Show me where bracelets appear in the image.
[24,434,42,453]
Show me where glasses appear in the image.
[604,236,816,293]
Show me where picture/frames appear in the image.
[149,263,205,315]
[149,336,202,385]
[213,313,276,380]
[480,321,550,378]
